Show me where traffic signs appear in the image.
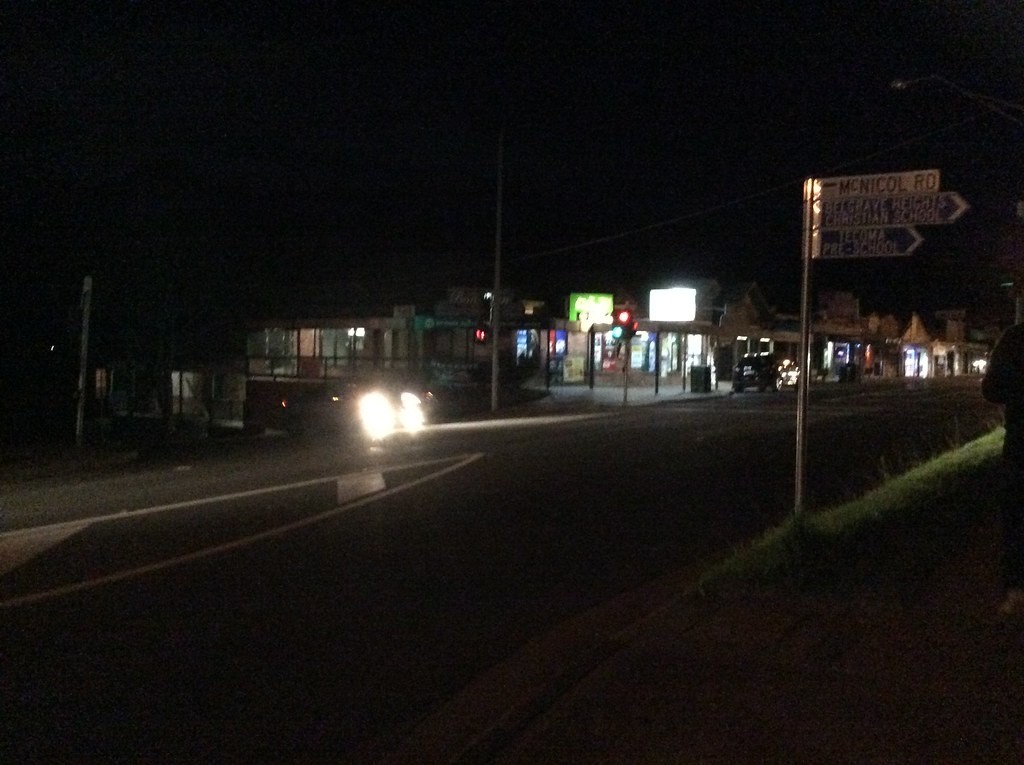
[813,190,973,232]
[811,226,926,259]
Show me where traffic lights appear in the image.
[473,326,489,345]
[611,309,639,340]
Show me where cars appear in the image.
[280,385,422,440]
[732,351,784,394]
